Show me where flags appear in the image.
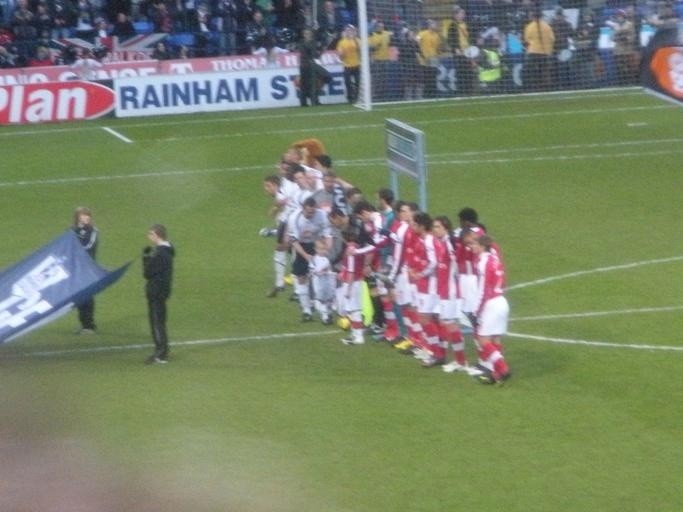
[0,228,138,344]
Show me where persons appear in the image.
[140,223,175,366]
[65,206,99,335]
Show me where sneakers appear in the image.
[145,355,171,364]
[72,326,96,335]
[265,275,511,387]
